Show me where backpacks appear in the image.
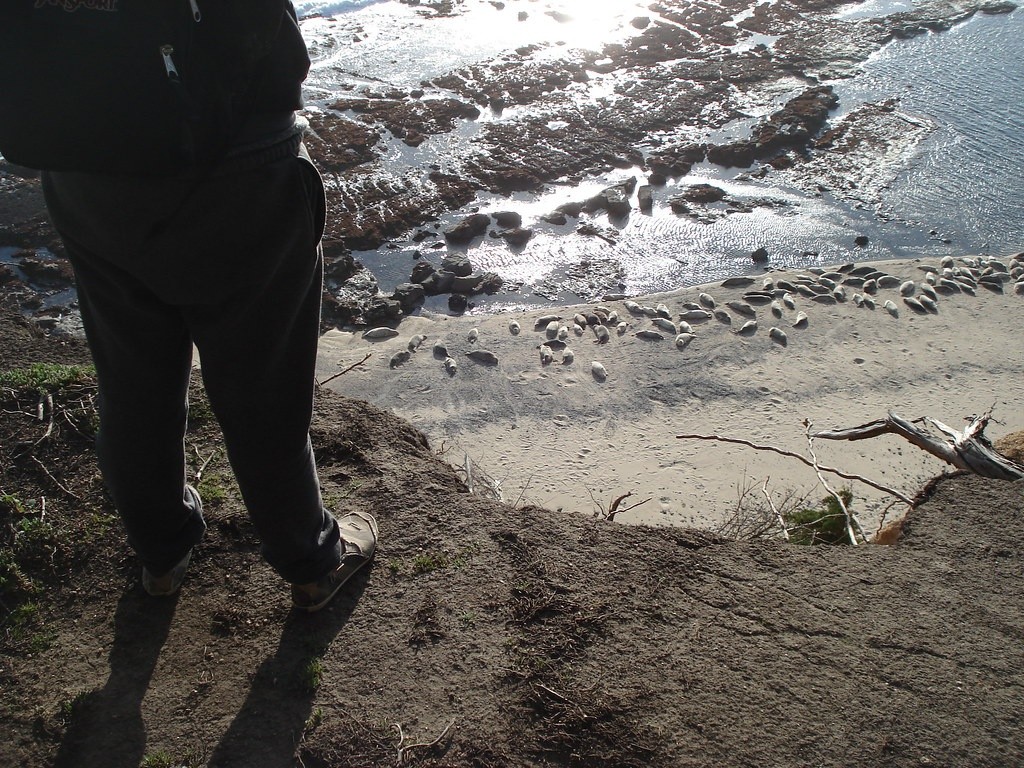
[0,0,286,180]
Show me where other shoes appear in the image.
[291,510,379,611]
[139,484,204,597]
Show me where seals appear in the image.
[352,251,1024,381]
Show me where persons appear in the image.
[0,0,379,609]
